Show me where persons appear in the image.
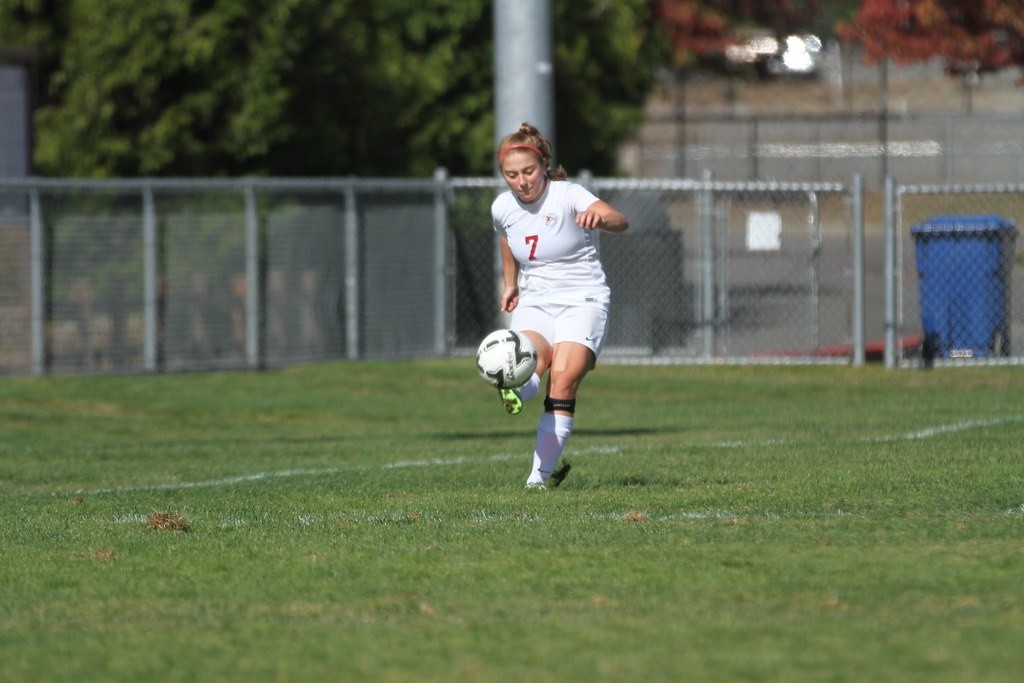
[492,122,630,493]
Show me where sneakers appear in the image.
[497,387,523,416]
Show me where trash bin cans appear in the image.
[910,215,1021,361]
[599,197,684,349]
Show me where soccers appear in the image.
[476,328,539,390]
